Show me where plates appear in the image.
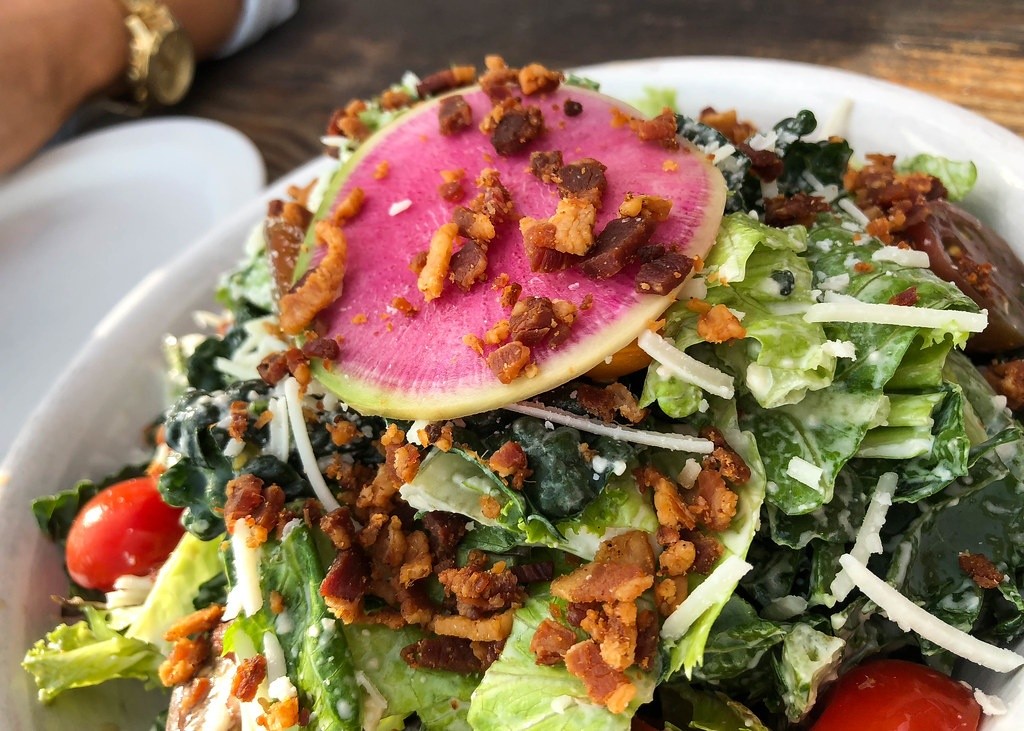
[1,54,1024,731]
[0,115,266,457]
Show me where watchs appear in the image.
[95,0,195,117]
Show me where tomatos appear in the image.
[810,659,982,731]
[66,478,184,590]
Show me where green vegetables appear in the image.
[20,81,1024,731]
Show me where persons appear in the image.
[0,0,314,180]
